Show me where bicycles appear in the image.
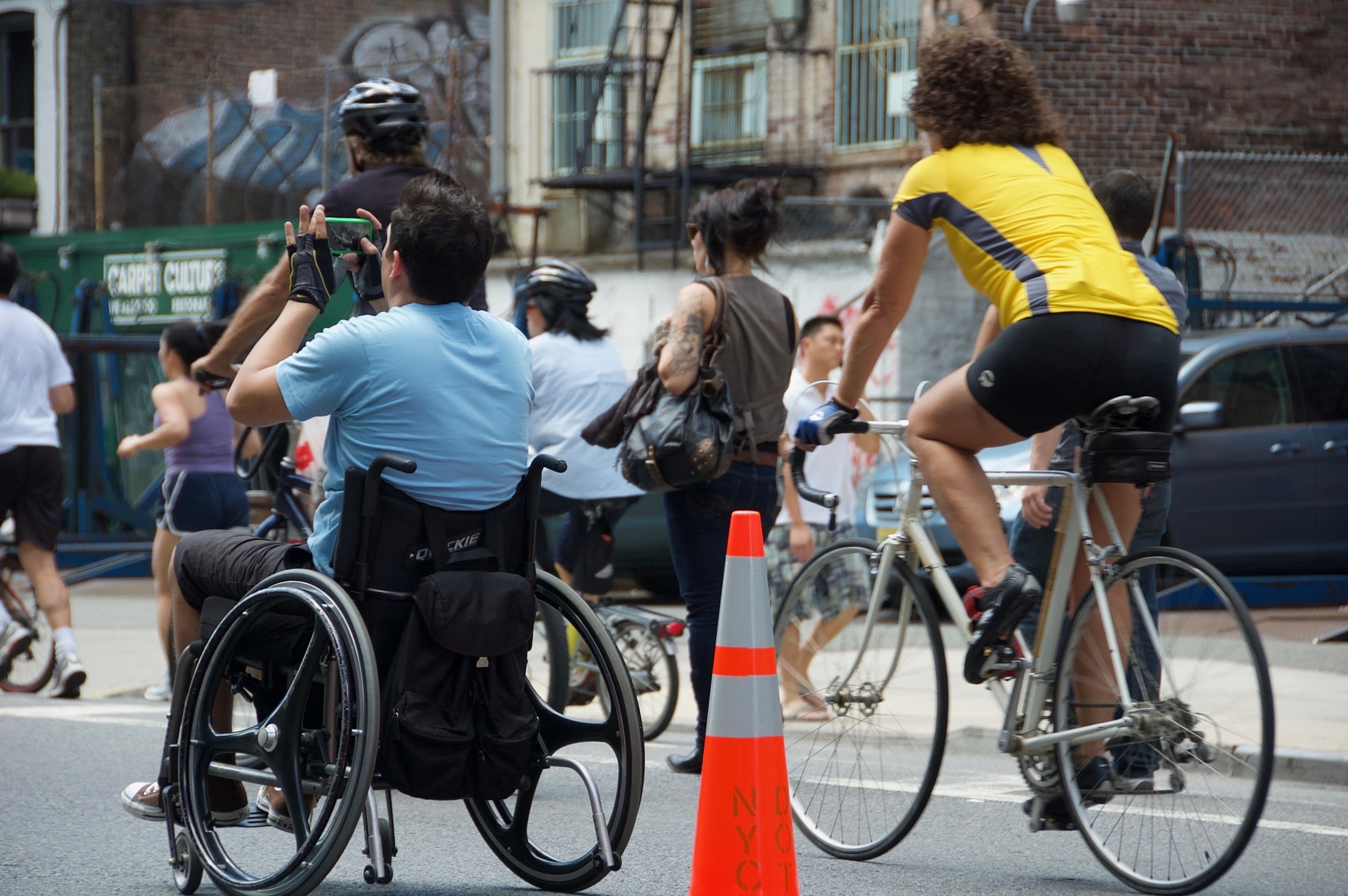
[774,380,1275,895]
[0,515,57,695]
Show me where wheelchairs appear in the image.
[155,447,646,896]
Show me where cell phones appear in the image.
[325,218,373,254]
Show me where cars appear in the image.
[855,323,1347,624]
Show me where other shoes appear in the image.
[1109,759,1153,791]
[0,621,32,681]
[144,683,173,702]
[48,652,87,698]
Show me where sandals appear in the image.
[797,684,827,711]
[783,706,830,721]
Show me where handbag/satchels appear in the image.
[621,276,734,494]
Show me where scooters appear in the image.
[526,491,691,742]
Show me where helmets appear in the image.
[516,261,597,305]
[338,78,429,135]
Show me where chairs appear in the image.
[1223,371,1280,427]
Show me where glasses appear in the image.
[686,223,702,238]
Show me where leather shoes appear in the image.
[665,748,703,772]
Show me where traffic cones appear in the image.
[689,509,798,896]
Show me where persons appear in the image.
[760,315,883,720]
[619,177,798,774]
[1,242,86,700]
[793,25,1180,832]
[508,257,634,707]
[120,177,535,834]
[1005,168,1205,791]
[187,77,492,381]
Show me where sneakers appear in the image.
[120,753,251,825]
[964,563,1043,684]
[1022,756,1115,819]
[255,785,314,834]
